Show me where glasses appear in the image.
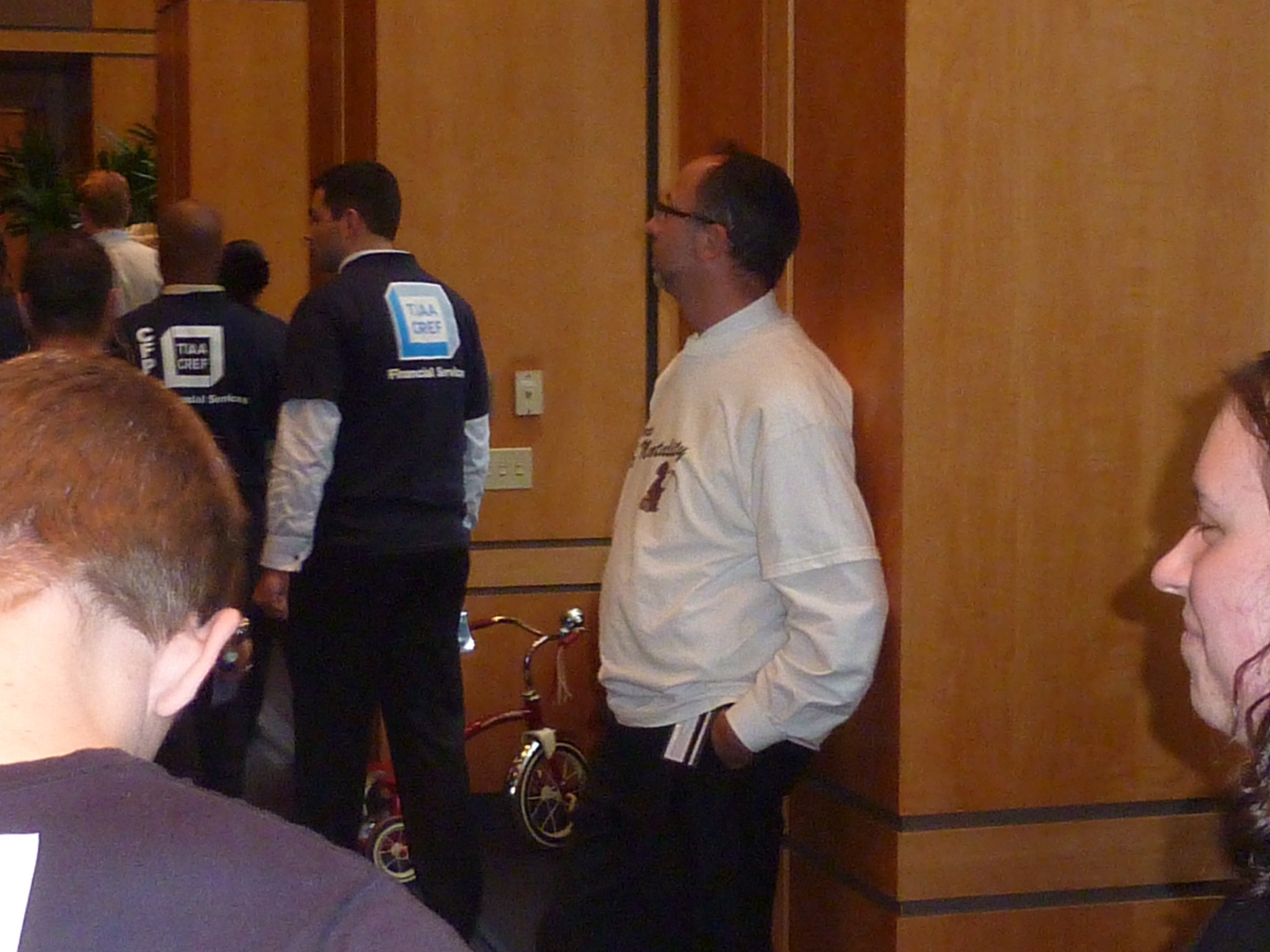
[653,200,735,247]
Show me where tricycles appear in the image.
[351,606,590,883]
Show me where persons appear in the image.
[108,200,288,796]
[1,349,472,952]
[0,237,29,360]
[1151,350,1270,952]
[18,231,120,353]
[252,162,493,941]
[218,237,269,308]
[586,143,889,952]
[78,169,164,318]
